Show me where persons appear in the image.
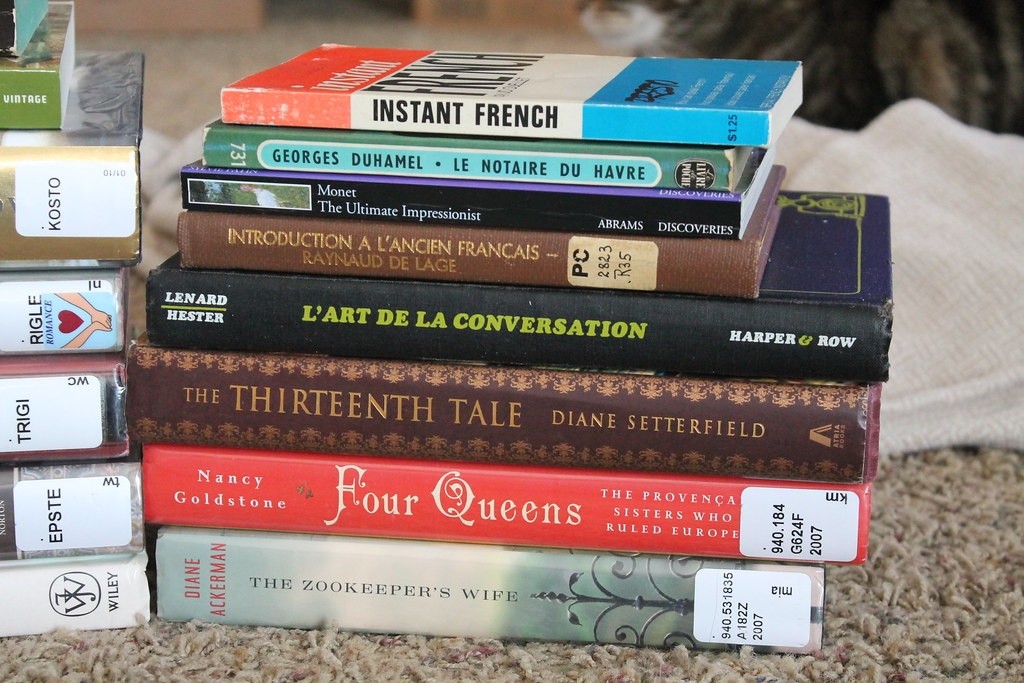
[661,0,1023,456]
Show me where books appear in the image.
[0,0,893,655]
